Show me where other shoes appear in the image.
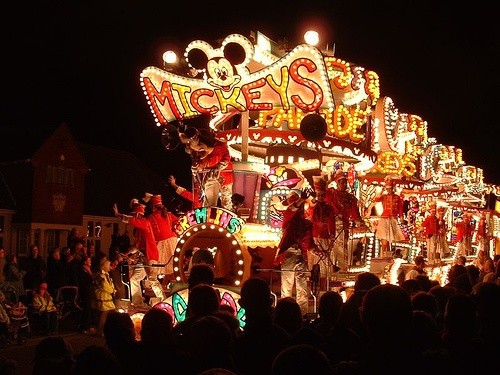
[97,333,104,337]
[131,302,145,308]
[82,326,97,334]
[52,331,59,335]
[42,330,51,335]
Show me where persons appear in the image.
[169,125,234,212]
[173,246,500,375]
[0,308,184,375]
[452,211,489,261]
[109,192,179,308]
[373,175,406,259]
[0,226,117,338]
[273,171,364,315]
[419,204,451,265]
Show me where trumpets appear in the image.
[315,239,341,275]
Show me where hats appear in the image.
[130,199,146,215]
[143,193,162,205]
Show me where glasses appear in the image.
[187,279,200,285]
